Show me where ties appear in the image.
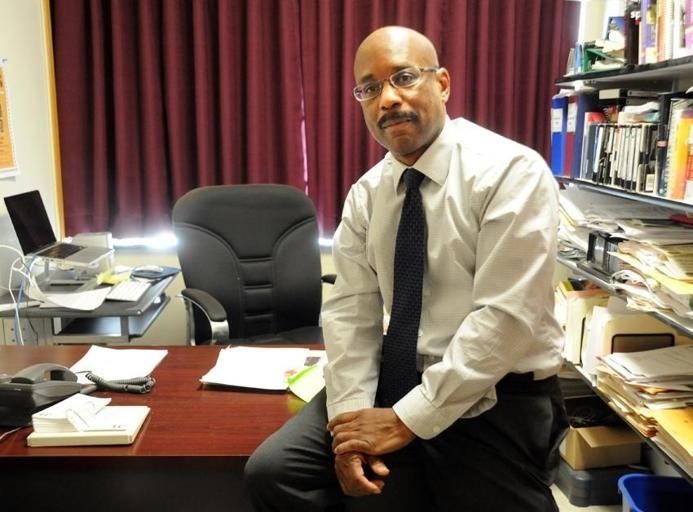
[377,169,425,406]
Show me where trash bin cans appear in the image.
[618,473,693,512]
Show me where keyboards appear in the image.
[105,279,151,302]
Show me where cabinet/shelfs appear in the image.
[554,54,693,486]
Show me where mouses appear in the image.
[136,264,162,272]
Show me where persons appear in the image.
[238,23,568,510]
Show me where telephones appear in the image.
[0,363,87,410]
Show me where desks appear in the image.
[0,342,326,470]
[0,272,179,345]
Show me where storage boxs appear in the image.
[557,418,647,471]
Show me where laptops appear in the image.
[3,190,114,266]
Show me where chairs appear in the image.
[171,184,336,346]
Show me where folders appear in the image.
[551,96,578,175]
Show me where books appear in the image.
[556,1,692,304]
[198,345,328,403]
[23,402,152,448]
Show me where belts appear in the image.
[416,355,441,374]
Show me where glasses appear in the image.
[353,65,436,103]
